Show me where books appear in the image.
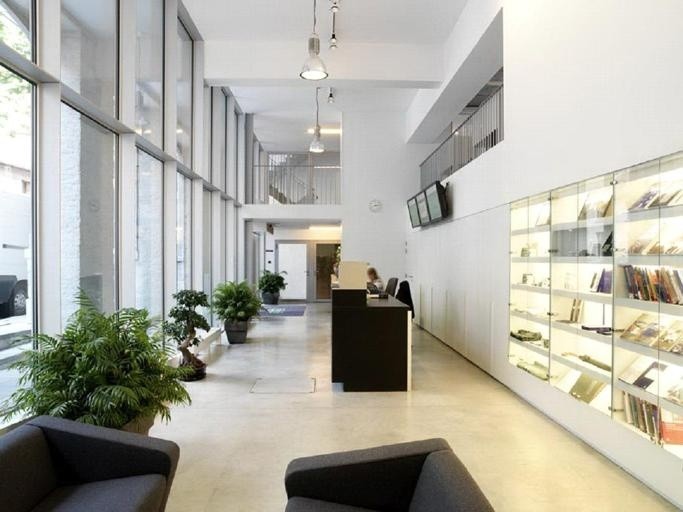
[549,187,683,447]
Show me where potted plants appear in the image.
[257,268,288,304]
[0,284,196,436]
[161,289,211,381]
[211,279,269,344]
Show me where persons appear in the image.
[367,268,382,290]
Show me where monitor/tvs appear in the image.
[406,195,422,229]
[414,189,431,226]
[423,179,448,223]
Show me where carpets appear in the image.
[258,304,307,317]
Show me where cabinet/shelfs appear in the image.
[508,149,683,462]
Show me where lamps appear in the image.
[299,0,338,152]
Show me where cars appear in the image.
[0,274,28,314]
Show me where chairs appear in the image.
[1,414,179,511]
[284,437,496,512]
[385,278,409,307]
